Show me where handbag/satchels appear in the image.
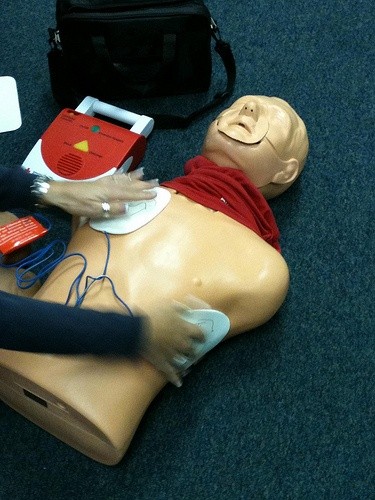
[46,0,237,130]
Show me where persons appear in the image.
[0,93,310,467]
[0,163,205,388]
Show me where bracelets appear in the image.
[31,175,52,206]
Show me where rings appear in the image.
[113,176,118,183]
[101,202,111,219]
